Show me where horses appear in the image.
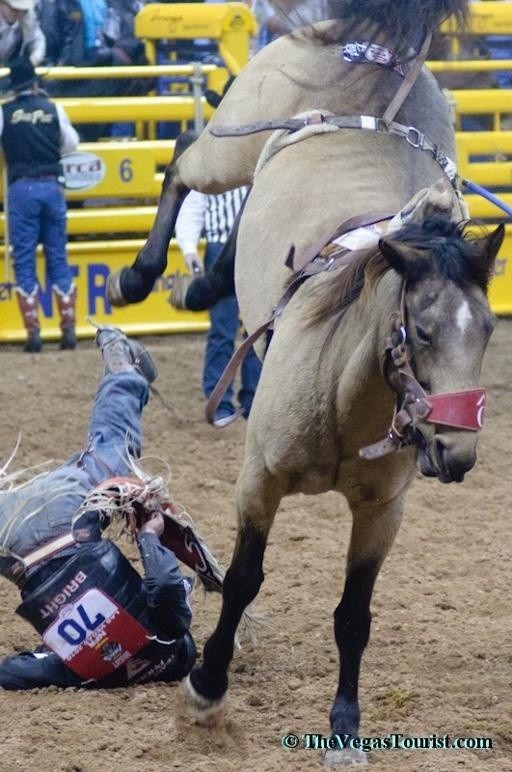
[101,0,508,771]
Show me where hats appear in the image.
[204,74,238,109]
[0,54,50,92]
[101,32,152,65]
[1,0,36,10]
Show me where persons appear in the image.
[0,325,225,689]
[2,55,78,353]
[173,71,277,429]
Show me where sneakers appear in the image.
[212,408,232,421]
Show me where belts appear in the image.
[21,532,75,569]
[16,175,58,182]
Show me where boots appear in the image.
[96,324,159,383]
[52,283,78,350]
[13,286,44,353]
